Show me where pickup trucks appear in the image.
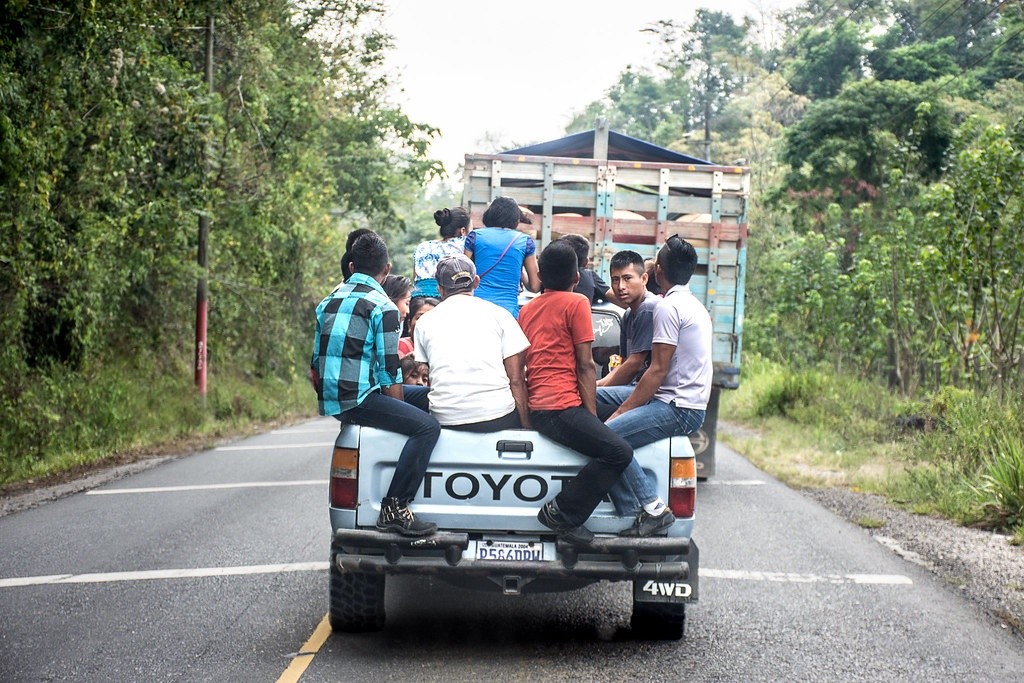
[327,301,700,640]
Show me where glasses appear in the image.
[665,233,678,252]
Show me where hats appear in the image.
[435,253,476,288]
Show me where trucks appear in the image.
[458,116,749,481]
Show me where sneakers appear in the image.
[617,506,675,537]
[376,497,437,536]
[537,503,595,545]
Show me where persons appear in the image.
[597,234,713,538]
[341,226,438,388]
[311,233,441,538]
[519,240,633,545]
[560,234,667,386]
[463,197,542,322]
[414,253,531,434]
[409,207,474,302]
